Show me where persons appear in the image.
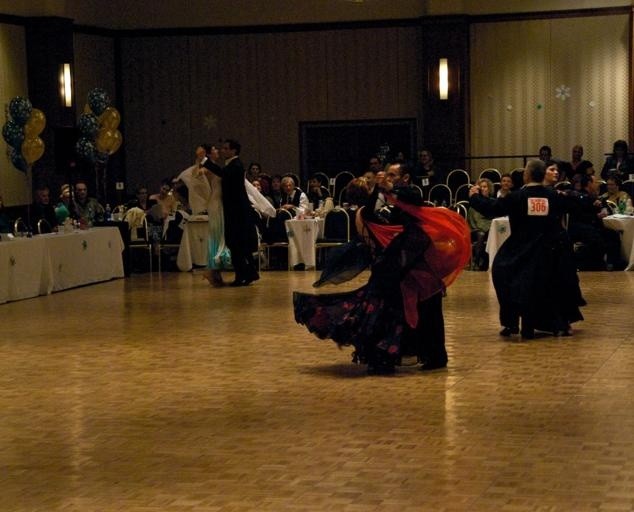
[412,147,444,206]
[395,149,408,161]
[600,139,634,181]
[268,174,283,208]
[360,167,376,192]
[274,176,309,269]
[147,177,174,211]
[466,177,496,271]
[372,158,448,371]
[539,146,551,162]
[304,174,328,211]
[366,156,383,173]
[194,138,260,286]
[292,174,472,377]
[572,173,628,271]
[493,172,514,198]
[247,162,270,193]
[28,184,58,234]
[171,143,232,287]
[250,178,274,208]
[559,144,594,182]
[66,182,106,226]
[126,183,164,267]
[599,175,634,216]
[53,183,76,226]
[167,176,181,195]
[569,174,583,195]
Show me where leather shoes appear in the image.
[500,326,519,336]
[521,333,541,340]
[228,276,245,287]
[418,362,447,371]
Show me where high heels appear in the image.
[202,269,227,287]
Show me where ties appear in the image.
[288,194,292,204]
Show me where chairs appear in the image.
[264,209,293,271]
[339,185,352,207]
[411,184,423,199]
[38,218,54,233]
[333,171,355,204]
[553,180,572,192]
[449,205,467,223]
[447,169,470,204]
[621,178,634,207]
[312,172,332,198]
[320,184,331,198]
[510,167,525,187]
[424,201,434,207]
[457,199,470,207]
[279,172,300,187]
[13,217,34,237]
[595,180,608,194]
[492,182,502,195]
[428,183,452,207]
[455,184,473,204]
[479,167,502,181]
[158,210,187,272]
[314,206,352,271]
[120,210,154,272]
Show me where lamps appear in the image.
[438,57,449,101]
[63,62,72,108]
